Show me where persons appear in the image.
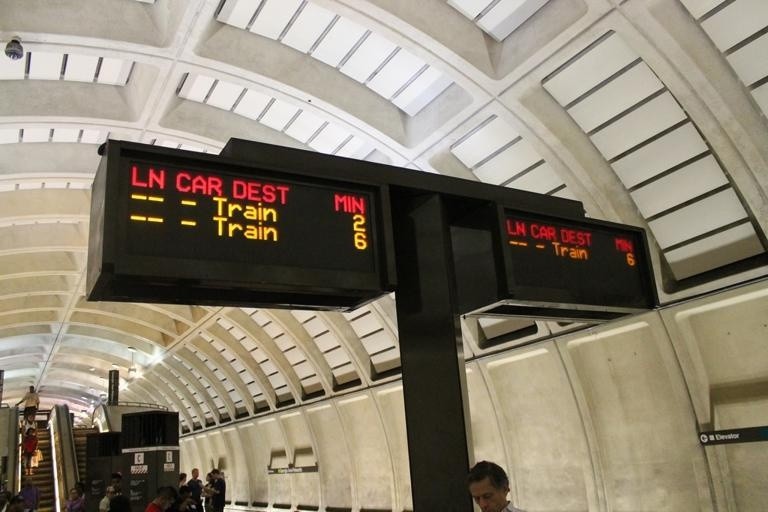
[63,466,226,512]
[464,460,524,512]
[0,385,41,512]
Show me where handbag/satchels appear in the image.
[31,449,43,467]
